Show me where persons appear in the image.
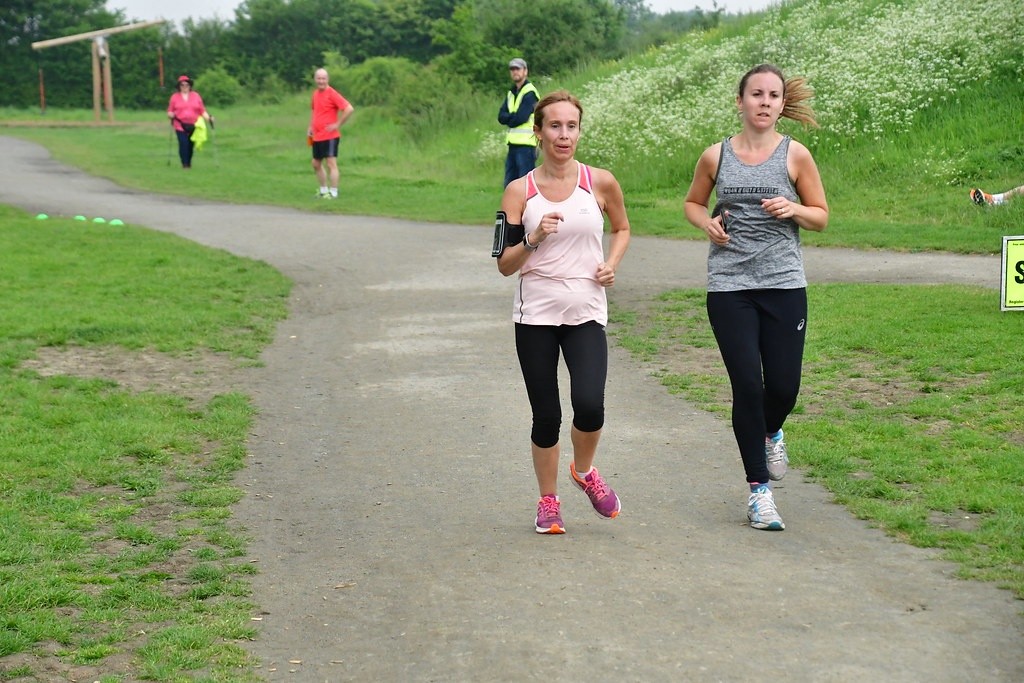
[491,92,629,534]
[683,64,828,531]
[970,185,1024,207]
[498,57,542,190]
[168,75,215,169]
[307,68,354,201]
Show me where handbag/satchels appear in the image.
[175,117,194,132]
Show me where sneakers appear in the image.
[747,486,785,530]
[766,429,789,480]
[569,462,621,519]
[535,494,566,534]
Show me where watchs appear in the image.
[523,233,541,254]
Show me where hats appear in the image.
[177,76,193,91]
[509,58,526,69]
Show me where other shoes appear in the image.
[323,192,333,199]
[315,192,323,198]
[970,188,993,206]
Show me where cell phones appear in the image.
[492,211,507,255]
[720,208,728,233]
